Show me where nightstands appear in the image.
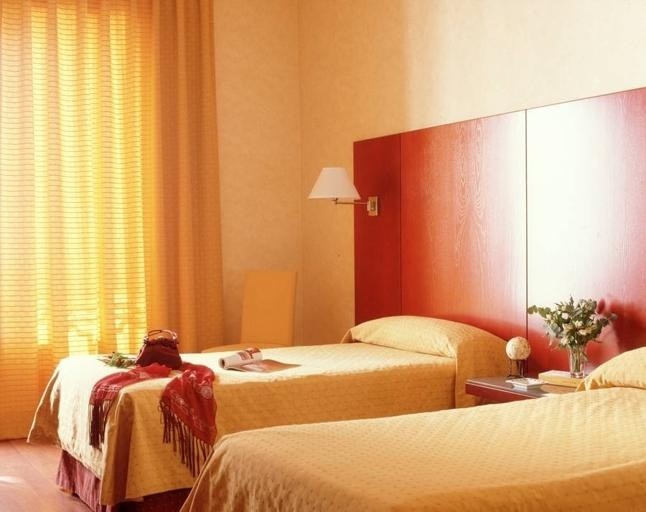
[463,372,578,406]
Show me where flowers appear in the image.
[525,299,618,352]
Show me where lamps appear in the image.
[308,167,379,217]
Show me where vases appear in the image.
[569,344,583,378]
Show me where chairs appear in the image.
[200,272,299,353]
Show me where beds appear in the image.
[23,314,519,512]
[178,344,646,512]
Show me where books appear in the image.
[504,378,547,387]
[538,370,586,388]
[217,347,301,373]
[511,384,539,390]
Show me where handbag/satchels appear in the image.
[135,330,181,370]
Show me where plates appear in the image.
[505,378,549,390]
